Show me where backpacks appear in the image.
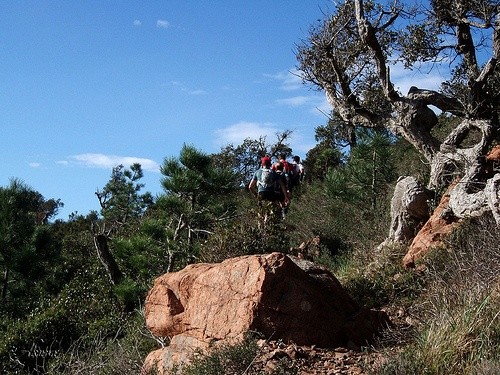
[290,162,303,184]
[276,161,291,185]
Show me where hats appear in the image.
[261,157,271,166]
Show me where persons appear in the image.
[274,163,290,219]
[271,153,290,198]
[249,157,290,232]
[287,156,303,194]
